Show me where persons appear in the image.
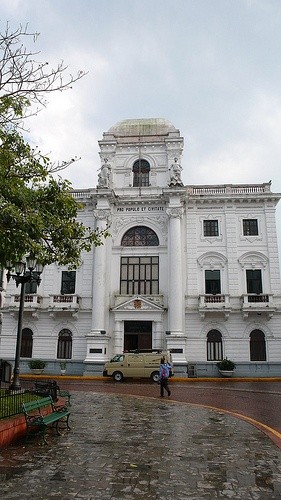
[97,174,105,185]
[169,157,183,183]
[97,157,112,187]
[158,357,170,397]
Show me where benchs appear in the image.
[20,395,71,446]
[32,380,71,407]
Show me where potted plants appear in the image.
[217,357,236,377]
[60,362,66,376]
[29,360,47,374]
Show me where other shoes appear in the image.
[168,390,171,396]
[160,394,164,397]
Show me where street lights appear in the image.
[4,250,44,390]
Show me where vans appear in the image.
[103,352,174,382]
